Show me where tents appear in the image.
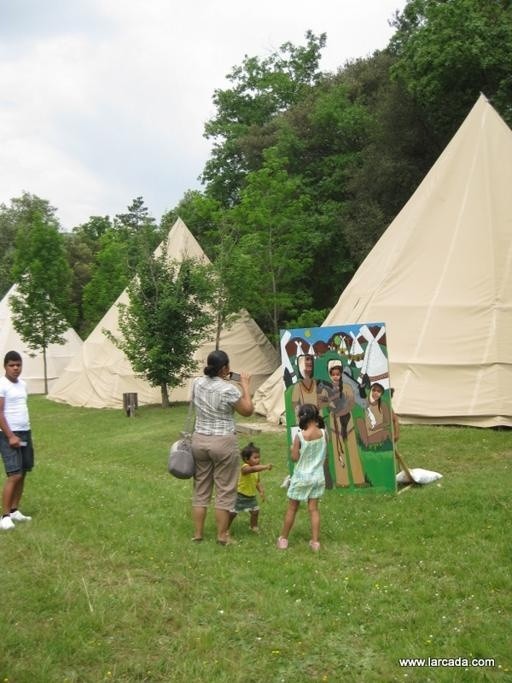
[247,92,512,429]
[45,216,280,409]
[0,267,83,395]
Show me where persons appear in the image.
[299,354,313,379]
[226,442,273,535]
[371,383,399,441]
[182,350,254,543]
[330,366,342,380]
[276,403,329,552]
[0,350,34,530]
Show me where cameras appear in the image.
[230,371,240,383]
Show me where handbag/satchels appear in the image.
[168,437,196,479]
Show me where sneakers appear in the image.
[309,539,320,551]
[10,510,32,521]
[0,516,15,531]
[277,536,288,549]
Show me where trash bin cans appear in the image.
[123,393,138,418]
